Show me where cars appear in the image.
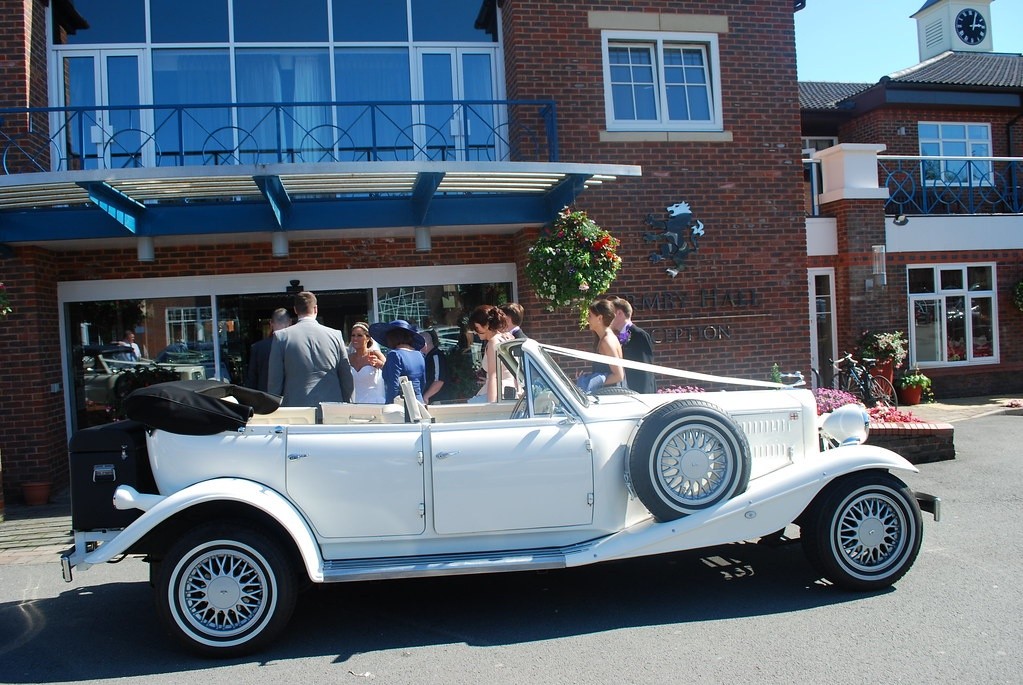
[57,339,944,662]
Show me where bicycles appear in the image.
[828,351,898,413]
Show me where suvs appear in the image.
[83,344,205,406]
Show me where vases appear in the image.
[870,364,894,397]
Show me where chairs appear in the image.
[398,375,433,422]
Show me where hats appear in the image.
[369,320,426,350]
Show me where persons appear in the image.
[609,297,658,394]
[498,303,529,339]
[344,321,387,405]
[584,299,630,389]
[423,318,439,348]
[267,291,354,407]
[112,330,142,369]
[457,319,475,364]
[368,319,427,405]
[465,304,521,402]
[245,306,292,392]
[418,332,449,404]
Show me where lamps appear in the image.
[864,244,886,292]
[272,231,289,256]
[416,226,431,252]
[138,235,155,262]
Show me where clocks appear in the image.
[955,7,986,45]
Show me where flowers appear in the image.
[617,327,632,347]
[527,206,624,330]
[853,330,909,369]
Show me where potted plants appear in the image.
[895,366,936,405]
